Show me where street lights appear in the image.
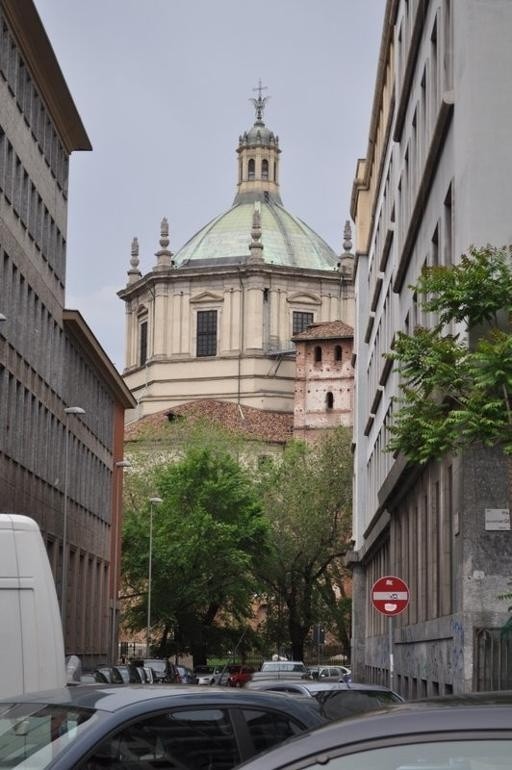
[143,498,164,659]
[111,461,131,665]
[61,407,86,650]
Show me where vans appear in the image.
[0,514,67,701]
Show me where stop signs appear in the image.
[370,576,410,617]
